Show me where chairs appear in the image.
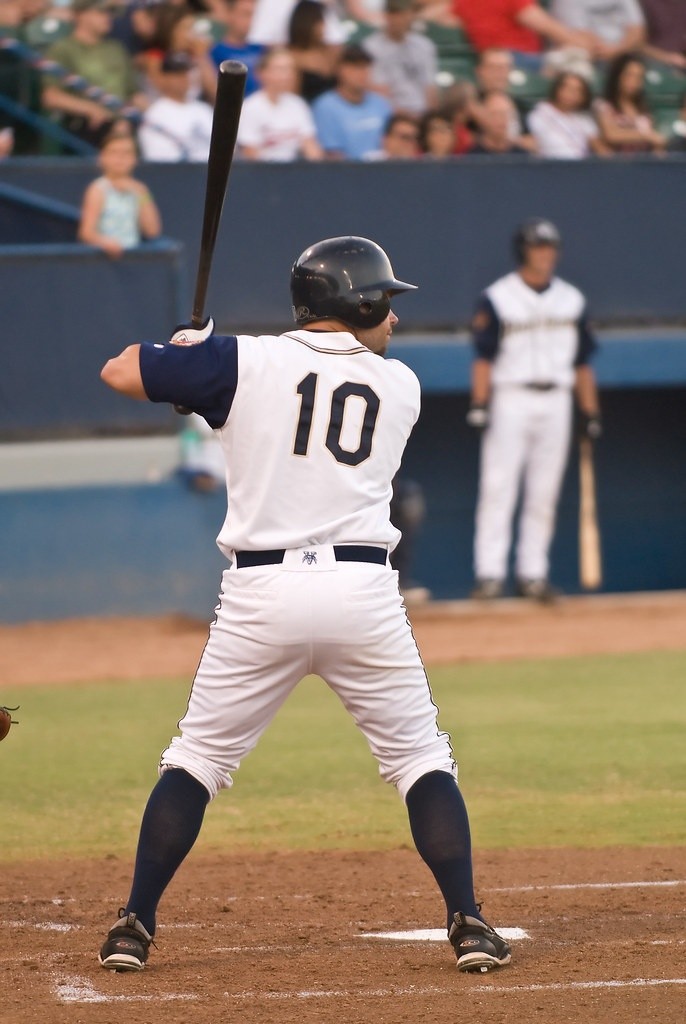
[341,20,686,156]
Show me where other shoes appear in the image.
[468,577,504,599]
[518,576,563,607]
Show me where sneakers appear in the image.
[444,911,512,972]
[98,912,154,972]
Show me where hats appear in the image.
[518,219,561,250]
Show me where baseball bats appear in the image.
[190,61,250,330]
[577,440,602,590]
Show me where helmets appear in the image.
[290,237,421,331]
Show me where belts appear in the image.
[234,545,390,568]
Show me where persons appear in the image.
[98,236,512,977]
[0,0,686,261]
[467,217,603,602]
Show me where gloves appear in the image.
[171,315,216,347]
[465,401,490,430]
[585,412,604,443]
